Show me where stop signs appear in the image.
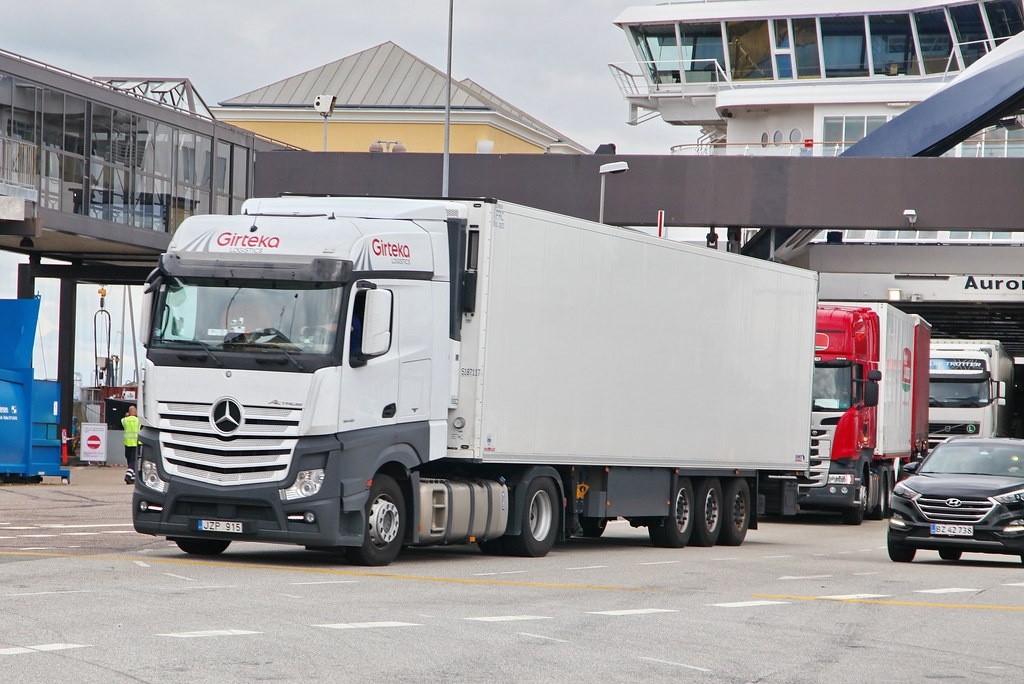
[86,435,101,450]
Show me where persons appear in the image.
[119,406,141,484]
[72,417,79,456]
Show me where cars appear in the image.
[885,434,1024,567]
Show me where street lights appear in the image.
[599,161,630,225]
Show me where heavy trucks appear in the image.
[132,191,821,567]
[926,338,1015,453]
[764,301,933,525]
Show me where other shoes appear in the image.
[131,480,136,483]
[124,477,131,485]
